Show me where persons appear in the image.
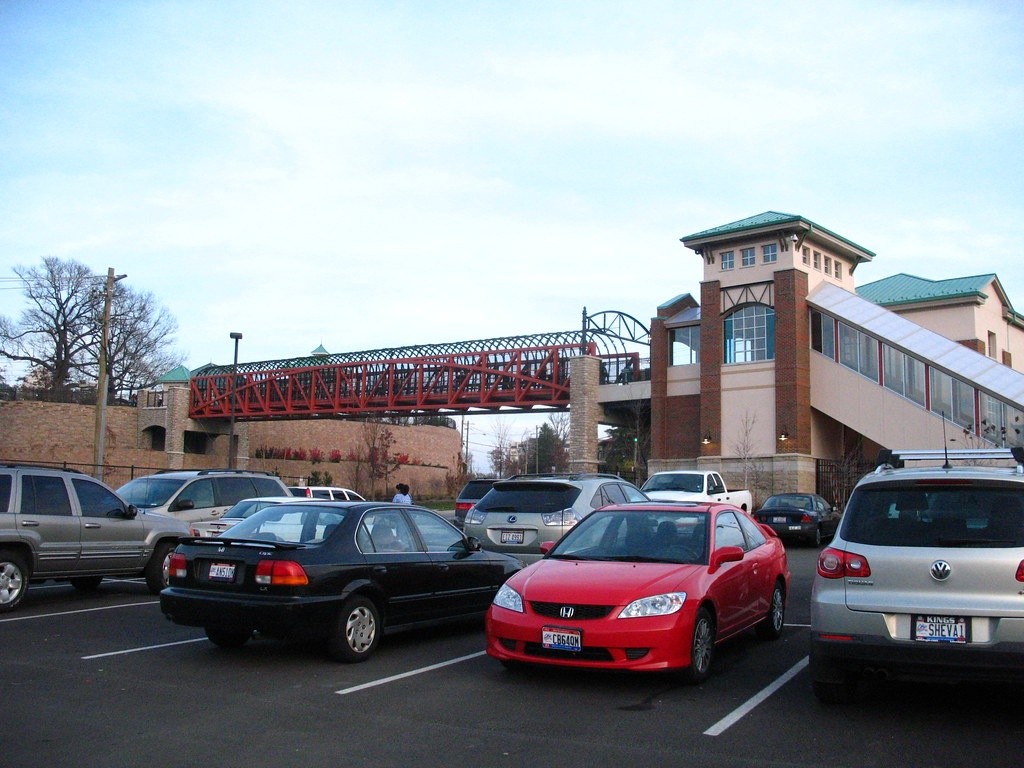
[392,483,415,505]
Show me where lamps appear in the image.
[778,424,790,441]
[701,431,712,444]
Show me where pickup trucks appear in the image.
[639,470,753,517]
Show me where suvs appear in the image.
[0,459,191,612]
[463,473,653,567]
[97,469,294,530]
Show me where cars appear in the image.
[287,487,366,501]
[754,491,842,547]
[486,500,791,682]
[161,499,530,662]
[811,411,1024,674]
[454,479,508,536]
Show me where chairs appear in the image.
[928,518,968,539]
[805,502,812,508]
[657,521,678,544]
[371,524,397,547]
[323,524,338,539]
[689,523,706,552]
[976,525,1024,538]
[779,502,789,506]
[887,518,935,537]
[624,524,651,546]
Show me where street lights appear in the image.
[228,332,242,471]
